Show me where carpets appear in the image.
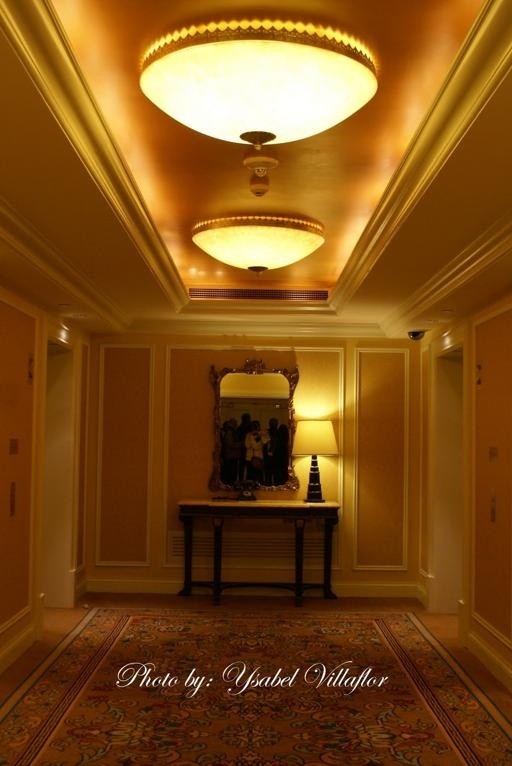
[1,608,511,765]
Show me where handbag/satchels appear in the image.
[251,456,264,469]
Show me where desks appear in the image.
[176,497,340,607]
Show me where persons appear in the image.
[221,414,289,486]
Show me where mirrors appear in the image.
[207,357,300,492]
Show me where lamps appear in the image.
[138,9,382,272]
[292,419,339,503]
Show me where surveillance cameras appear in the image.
[407,331,424,340]
